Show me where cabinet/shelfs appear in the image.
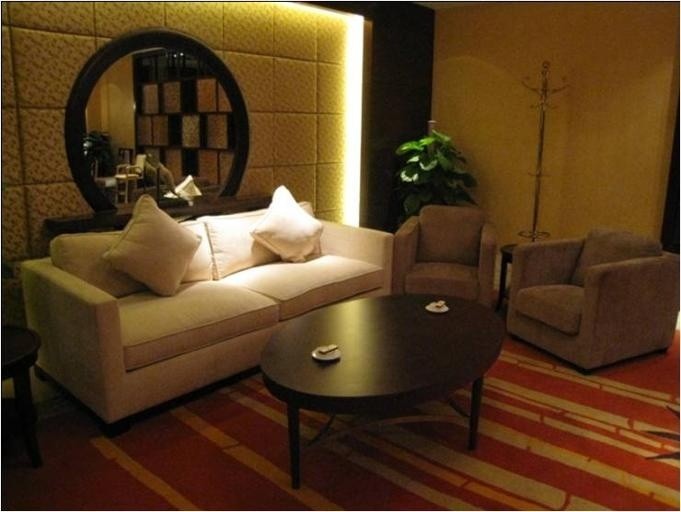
[361,0,437,231]
[44,196,279,237]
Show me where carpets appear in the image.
[1,332,679,512]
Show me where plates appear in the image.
[311,347,342,362]
[425,304,450,314]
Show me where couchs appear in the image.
[502,228,680,374]
[21,202,395,426]
[388,203,502,314]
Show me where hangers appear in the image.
[518,59,569,245]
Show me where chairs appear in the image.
[95,148,134,191]
[114,154,148,205]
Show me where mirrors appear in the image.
[62,28,251,212]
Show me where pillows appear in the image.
[249,185,325,264]
[99,192,201,297]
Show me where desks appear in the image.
[0,324,43,471]
[500,243,518,310]
